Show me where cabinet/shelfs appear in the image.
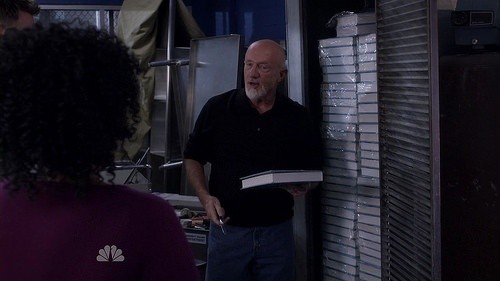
[374,1,500,281]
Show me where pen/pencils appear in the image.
[219,217,228,234]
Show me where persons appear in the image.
[1,17,200,281]
[183,39,330,281]
[1,0,41,36]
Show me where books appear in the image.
[238,168,324,192]
[316,12,383,281]
